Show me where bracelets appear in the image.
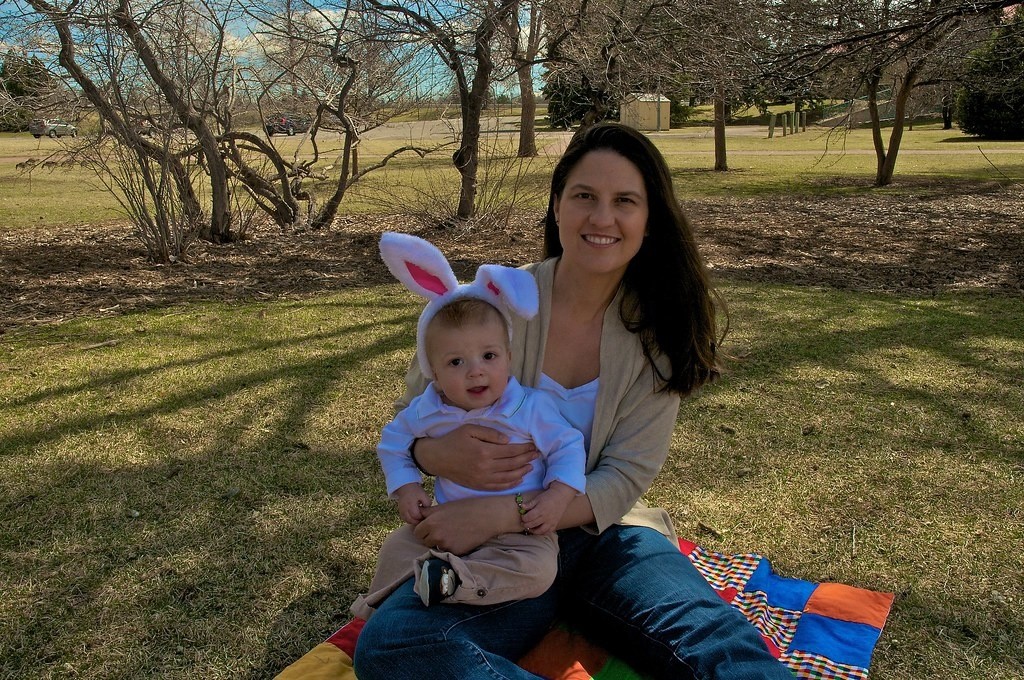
[515,493,529,531]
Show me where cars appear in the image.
[135,113,184,138]
[262,111,314,135]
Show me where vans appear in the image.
[29,118,78,138]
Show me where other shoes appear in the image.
[419,556,455,608]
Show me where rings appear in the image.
[436,545,439,550]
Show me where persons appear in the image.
[350,231,586,622]
[353,122,799,680]
